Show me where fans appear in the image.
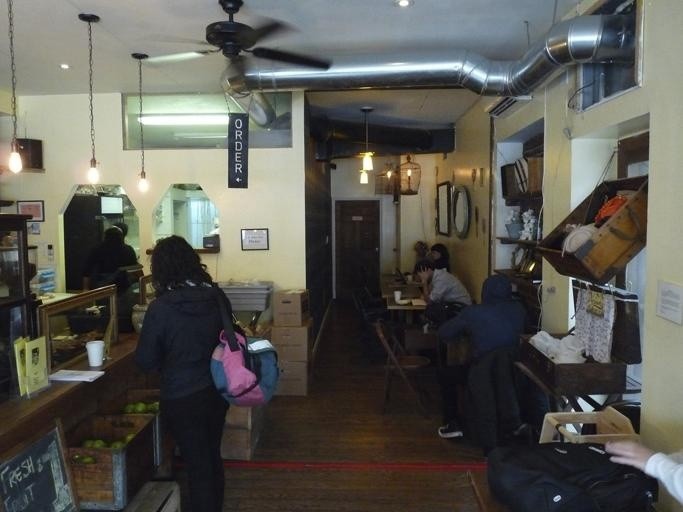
[145,0,330,72]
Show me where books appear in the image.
[407,298,427,306]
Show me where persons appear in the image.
[429,243,451,271]
[412,259,474,330]
[134,236,235,512]
[113,222,128,239]
[437,272,532,442]
[87,225,137,337]
[604,438,682,508]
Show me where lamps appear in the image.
[359,106,375,170]
[75,12,99,187]
[132,52,149,195]
[373,161,425,198]
[7,0,23,174]
[359,112,368,184]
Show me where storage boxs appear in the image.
[269,318,312,362]
[220,428,261,462]
[226,405,263,429]
[272,289,308,326]
[272,362,307,398]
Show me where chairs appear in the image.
[352,268,526,444]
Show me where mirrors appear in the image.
[451,185,470,240]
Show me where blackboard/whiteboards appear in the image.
[0,427,76,512]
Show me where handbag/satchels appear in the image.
[211,324,279,406]
[488,442,658,512]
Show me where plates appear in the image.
[395,300,409,305]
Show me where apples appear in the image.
[126,402,159,413]
[74,433,136,463]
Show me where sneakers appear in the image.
[438,424,464,438]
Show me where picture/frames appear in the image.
[39,282,120,377]
[16,200,44,222]
[436,181,449,236]
[240,228,269,251]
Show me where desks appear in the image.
[514,359,641,445]
[468,459,658,511]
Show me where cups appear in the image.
[85,341,105,367]
[393,290,401,302]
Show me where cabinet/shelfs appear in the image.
[496,133,542,311]
[1,211,43,399]
